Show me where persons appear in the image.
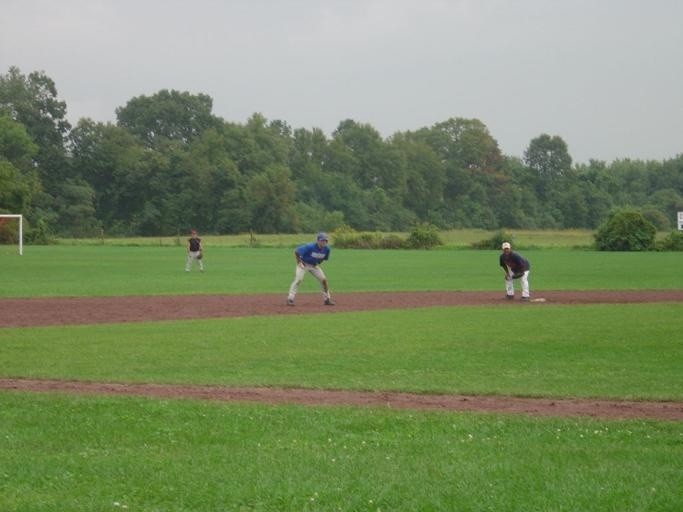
[287,232,336,306]
[185,230,203,272]
[499,242,530,301]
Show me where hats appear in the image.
[317,233,328,241]
[502,242,510,250]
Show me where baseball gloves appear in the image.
[512,272,524,278]
[196,254,202,259]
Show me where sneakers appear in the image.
[521,297,529,301]
[325,300,335,305]
[503,295,513,300]
[287,298,294,306]
